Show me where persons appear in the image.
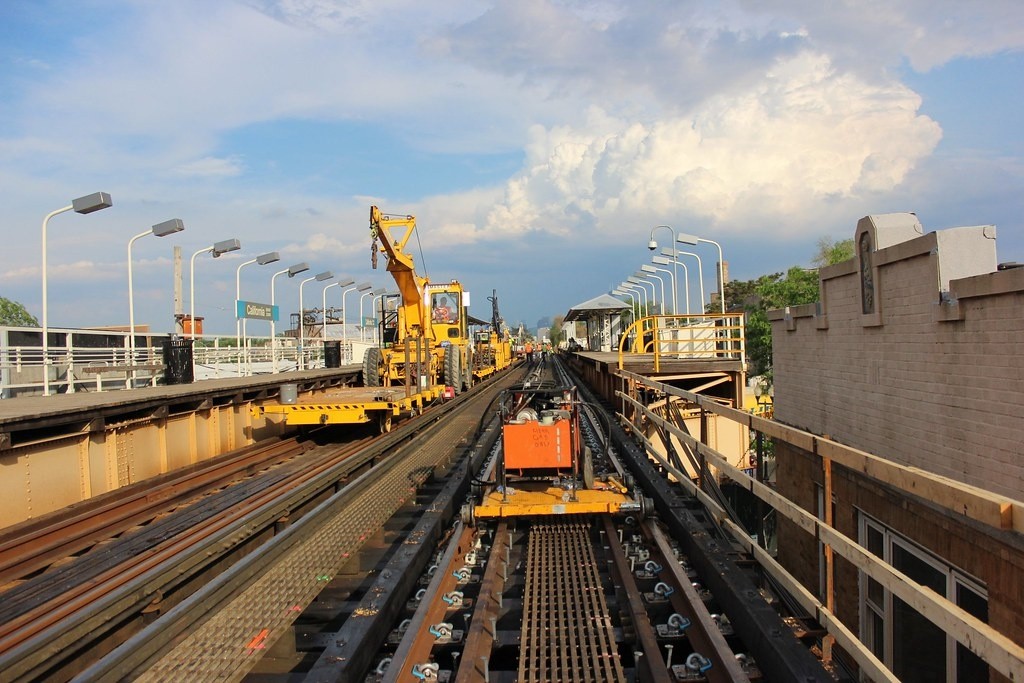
[537,343,551,352]
[568,338,575,352]
[481,335,487,340]
[541,343,546,362]
[525,342,533,363]
[434,298,451,323]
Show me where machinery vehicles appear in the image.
[248,203,520,436]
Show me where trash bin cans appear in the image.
[323,341,341,368]
[160,340,194,385]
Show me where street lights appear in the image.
[651,256,690,323]
[648,224,677,327]
[323,276,356,341]
[616,285,641,319]
[189,238,241,381]
[627,275,656,315]
[298,271,334,372]
[270,261,311,373]
[373,289,401,343]
[677,231,727,358]
[127,218,185,388]
[43,192,113,397]
[234,250,280,376]
[641,264,677,327]
[621,281,649,329]
[660,245,705,322]
[359,286,387,342]
[342,282,372,367]
[610,290,635,334]
[633,271,666,315]
